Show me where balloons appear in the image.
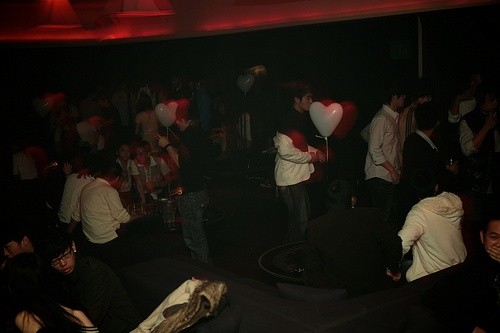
[154,98,191,131]
[309,94,357,141]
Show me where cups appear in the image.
[161,199,176,224]
[122,201,157,216]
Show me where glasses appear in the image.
[49,247,72,268]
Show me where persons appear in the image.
[361,82,500,333]
[271,84,326,254]
[16,102,213,269]
[0,220,142,333]
